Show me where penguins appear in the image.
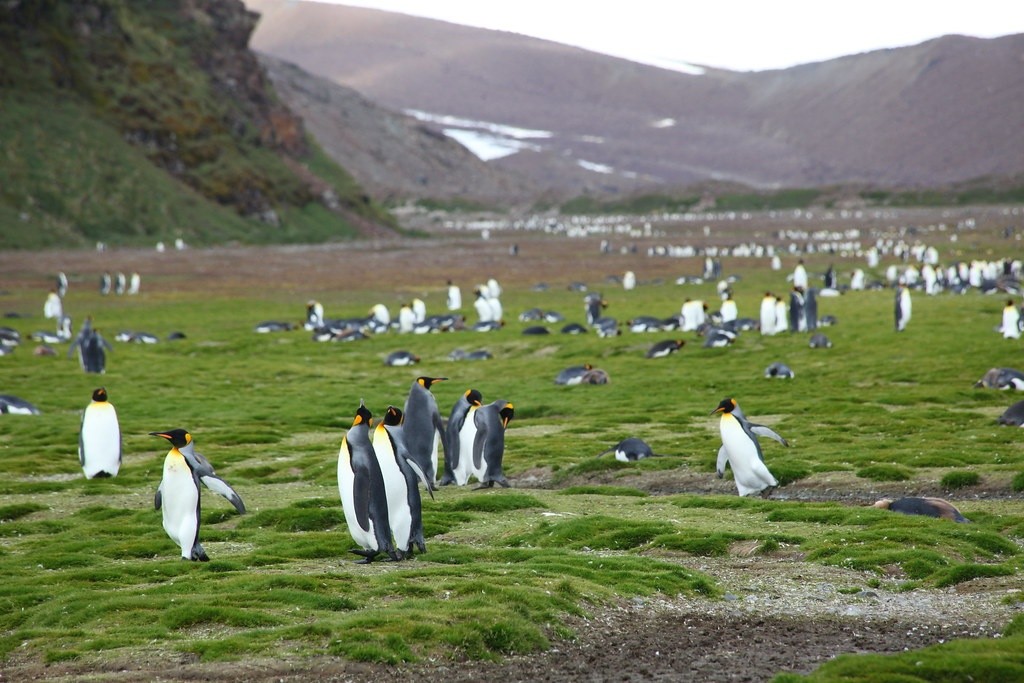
[337,398,399,564]
[710,397,789,499]
[0,208,1024,428]
[869,496,970,524]
[373,405,438,563]
[439,388,483,489]
[595,439,665,463]
[75,388,125,482]
[402,376,451,495]
[469,400,516,491]
[148,426,248,562]
